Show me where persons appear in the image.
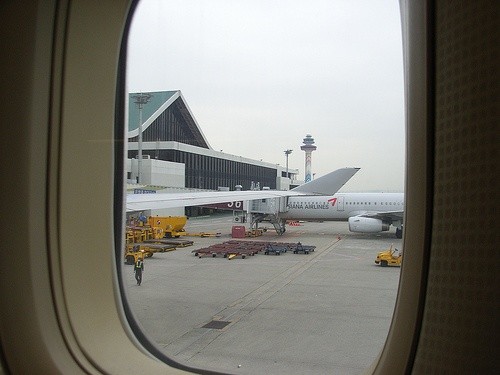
[133,256,145,287]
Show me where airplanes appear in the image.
[126,166,405,240]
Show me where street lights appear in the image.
[132,92,153,184]
[283,150,293,179]
